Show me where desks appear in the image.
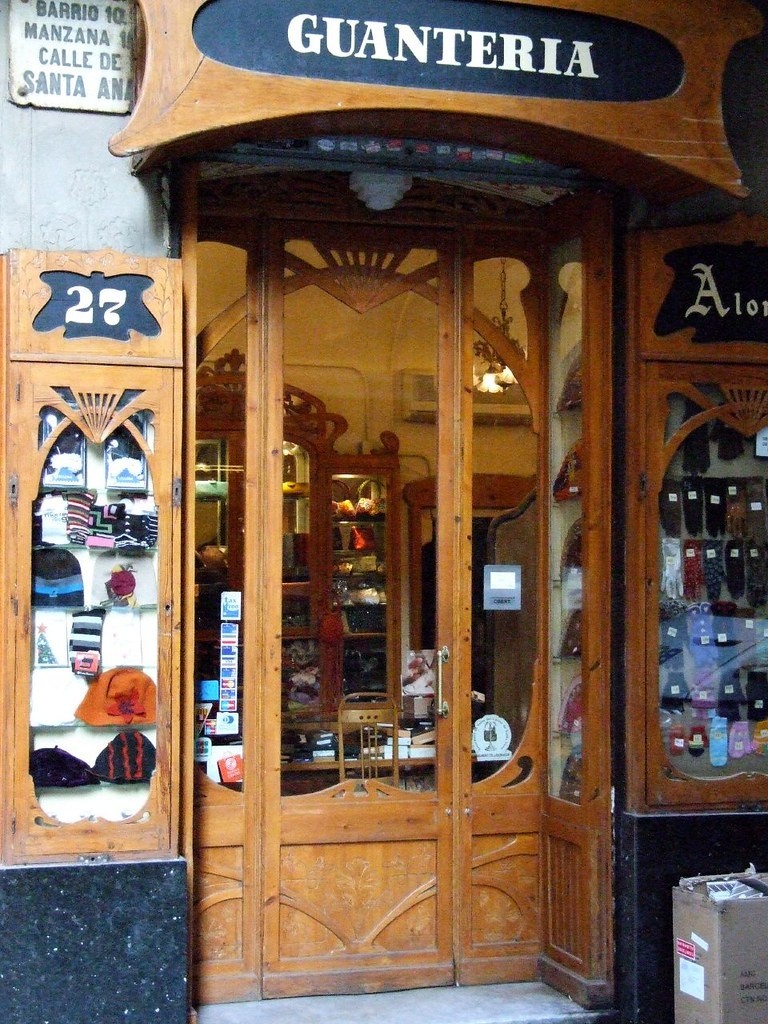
[199,756,506,796]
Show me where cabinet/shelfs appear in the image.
[536,187,616,1007]
[194,347,402,724]
[612,210,768,815]
[0,248,184,866]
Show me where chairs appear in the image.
[337,690,400,789]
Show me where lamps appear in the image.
[472,257,529,393]
[349,128,412,211]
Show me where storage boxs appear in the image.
[672,873,768,1024]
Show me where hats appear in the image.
[28,745,102,787]
[74,666,156,726]
[85,730,156,783]
[32,548,84,607]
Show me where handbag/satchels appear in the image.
[193,477,388,583]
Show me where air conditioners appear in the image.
[393,366,531,426]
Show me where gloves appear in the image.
[43,404,84,476]
[105,414,144,479]
[659,398,768,767]
